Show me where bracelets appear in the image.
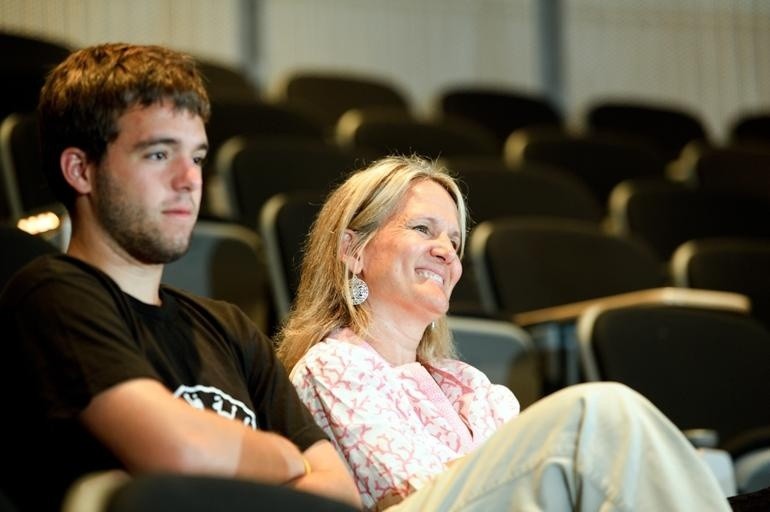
[303,456,312,473]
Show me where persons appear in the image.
[273,144,737,512]
[1,42,379,511]
